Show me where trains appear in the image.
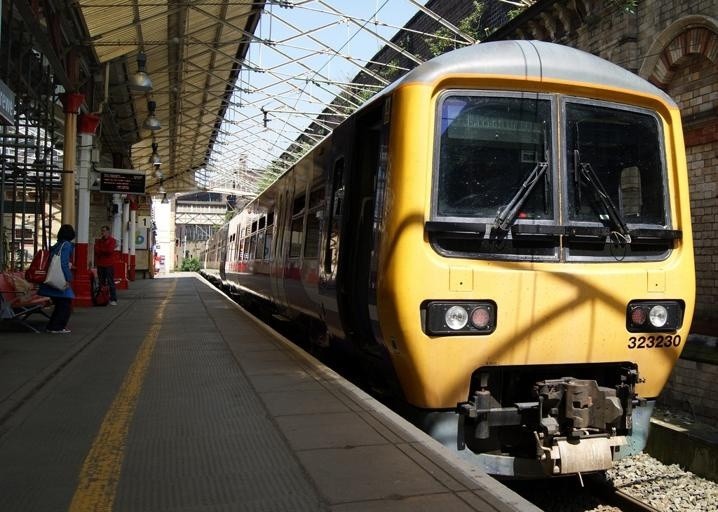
[195,37,699,482]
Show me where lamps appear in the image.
[141,101,161,130]
[149,144,162,164]
[161,194,169,203]
[124,53,153,91]
[151,165,163,179]
[157,180,166,193]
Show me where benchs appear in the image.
[0,271,52,334]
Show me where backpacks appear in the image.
[8,274,33,302]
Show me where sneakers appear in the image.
[109,300,117,306]
[46,325,72,334]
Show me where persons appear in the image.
[93,225,118,306]
[35,223,77,334]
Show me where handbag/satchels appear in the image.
[25,249,50,283]
[94,285,110,305]
[42,254,73,291]
